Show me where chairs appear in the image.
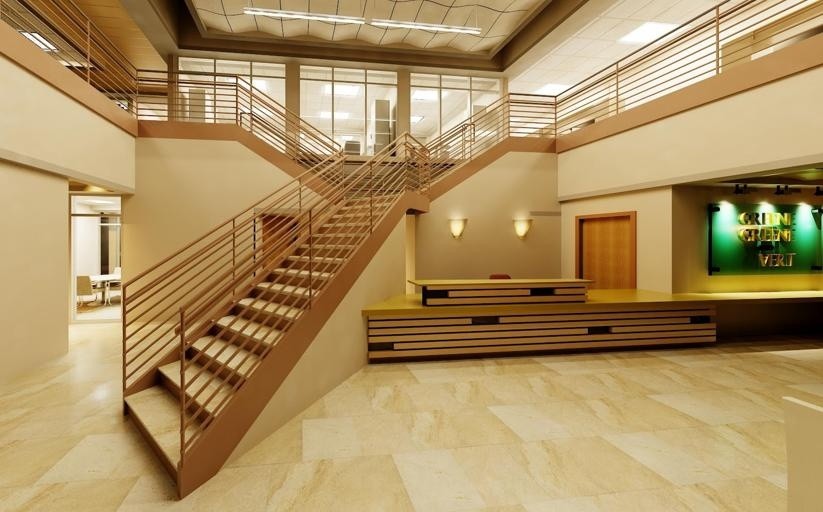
[77,276,96,308]
[489,274,511,279]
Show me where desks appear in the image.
[91,274,121,307]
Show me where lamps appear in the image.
[714,183,823,197]
